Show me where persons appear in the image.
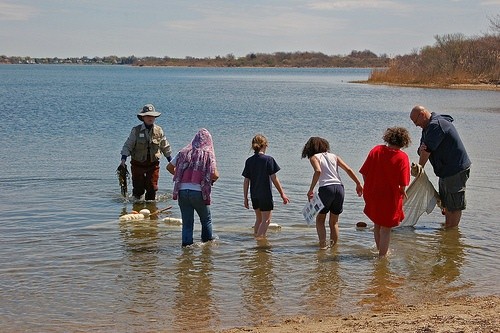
[359,128,412,256]
[121,104,172,201]
[242,135,291,237]
[410,106,472,227]
[301,137,363,247]
[167,129,219,246]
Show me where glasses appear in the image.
[414,113,421,124]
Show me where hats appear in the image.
[137,104,162,121]
